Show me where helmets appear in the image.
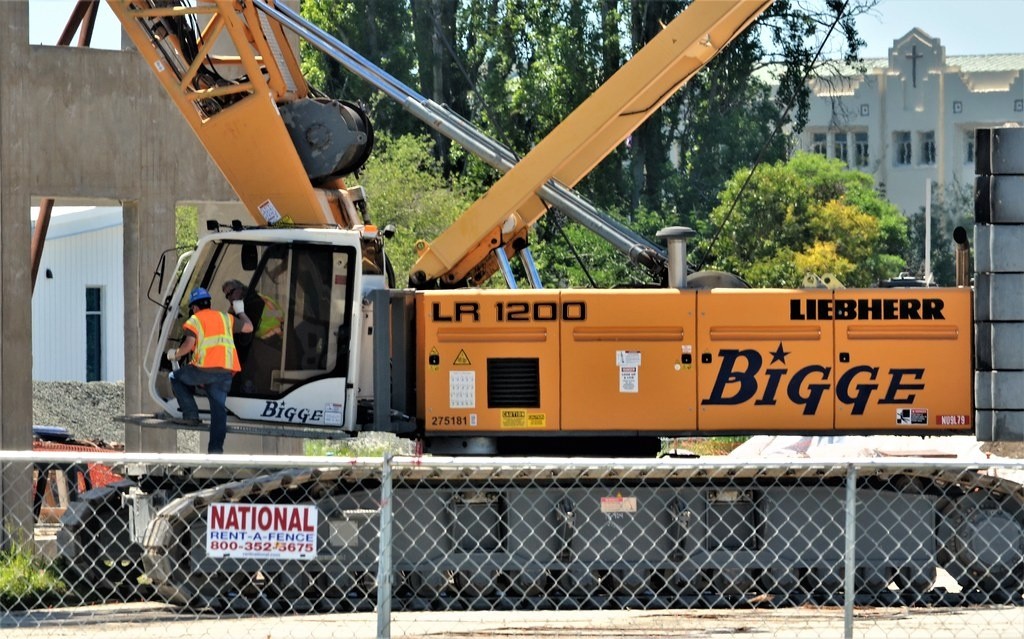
[189,287,211,304]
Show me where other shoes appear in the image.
[171,417,199,426]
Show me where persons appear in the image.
[167,288,254,454]
[221,280,303,391]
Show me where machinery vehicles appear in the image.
[52,0,1024,610]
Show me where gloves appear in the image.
[233,300,244,314]
[167,349,175,360]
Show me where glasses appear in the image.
[226,288,234,299]
